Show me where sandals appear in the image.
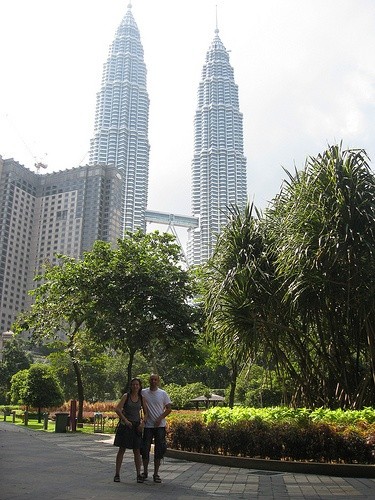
[141,473,148,481]
[153,474,161,483]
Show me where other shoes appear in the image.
[114,476,120,481]
[137,476,144,483]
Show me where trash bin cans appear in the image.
[55,413,69,433]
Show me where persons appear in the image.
[114,378,149,483]
[141,374,172,483]
